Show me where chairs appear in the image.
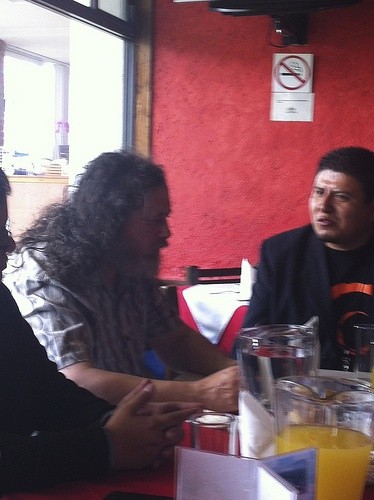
[186,265,258,285]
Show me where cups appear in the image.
[187,412,239,456]
[353,323,374,382]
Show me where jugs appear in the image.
[273,377,374,500]
[236,325,320,417]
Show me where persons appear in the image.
[0,168,203,499]
[1,149,247,415]
[230,146,373,378]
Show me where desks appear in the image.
[0,368,373,499]
[175,283,256,356]
